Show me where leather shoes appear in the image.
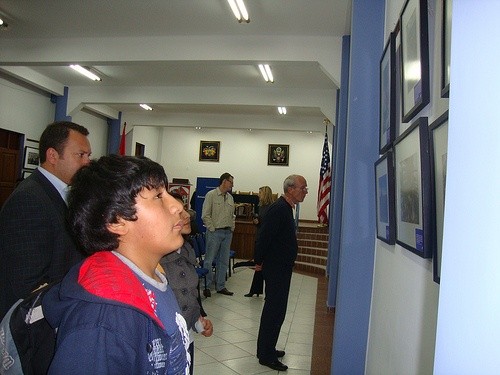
[216,287,234,296]
[258,355,289,372]
[257,348,286,359]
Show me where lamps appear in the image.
[259,64,274,83]
[228,0,250,24]
[70,64,102,81]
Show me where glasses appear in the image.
[227,179,233,185]
[300,186,309,194]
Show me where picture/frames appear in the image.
[391,116,432,259]
[24,146,40,170]
[398,0,430,123]
[440,0,452,98]
[378,32,396,154]
[427,109,448,282]
[374,150,396,246]
[199,140,220,162]
[23,171,32,179]
[268,144,289,166]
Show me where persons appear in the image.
[200,172,234,298]
[244,186,273,298]
[1,121,94,307]
[49,156,191,375]
[156,189,213,375]
[251,174,309,370]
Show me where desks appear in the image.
[232,194,259,219]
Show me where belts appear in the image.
[216,226,232,230]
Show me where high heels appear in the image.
[244,290,261,298]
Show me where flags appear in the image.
[315,131,333,227]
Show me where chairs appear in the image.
[237,191,259,220]
[187,232,235,299]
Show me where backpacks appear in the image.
[0,269,67,375]
